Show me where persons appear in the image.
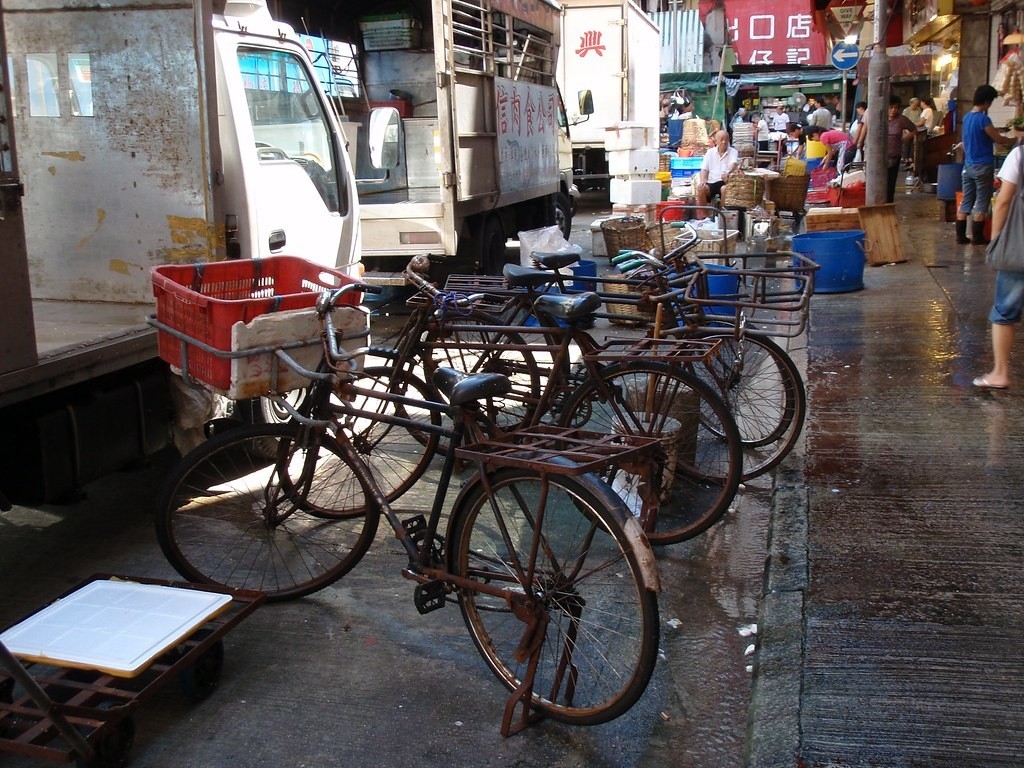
[737,96,869,178]
[887,95,933,202]
[972,143,1024,389]
[704,116,720,146]
[955,86,1019,245]
[696,130,739,221]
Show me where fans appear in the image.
[792,92,806,107]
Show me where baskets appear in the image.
[600,217,646,263]
[646,220,681,255]
[600,273,654,324]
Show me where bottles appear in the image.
[792,231,867,294]
[656,263,737,333]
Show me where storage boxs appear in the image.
[148,253,362,390]
[675,229,740,265]
[359,101,414,118]
[588,125,704,257]
[358,12,422,52]
[732,123,753,146]
[805,208,862,231]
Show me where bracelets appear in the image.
[857,141,861,143]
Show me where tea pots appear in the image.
[905,171,916,186]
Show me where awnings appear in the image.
[855,54,932,78]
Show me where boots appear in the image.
[972,220,990,245]
[955,220,970,244]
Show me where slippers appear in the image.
[971,373,1007,388]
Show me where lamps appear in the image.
[1002,24,1024,44]
[908,45,915,55]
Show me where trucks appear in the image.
[0,0,403,547]
[548,0,664,202]
[266,0,595,306]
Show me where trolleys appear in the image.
[770,136,805,217]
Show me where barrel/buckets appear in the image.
[520,260,597,329]
[936,165,965,202]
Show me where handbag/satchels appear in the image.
[984,143,1024,271]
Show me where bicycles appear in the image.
[389,198,821,488]
[152,280,663,737]
[388,249,744,561]
